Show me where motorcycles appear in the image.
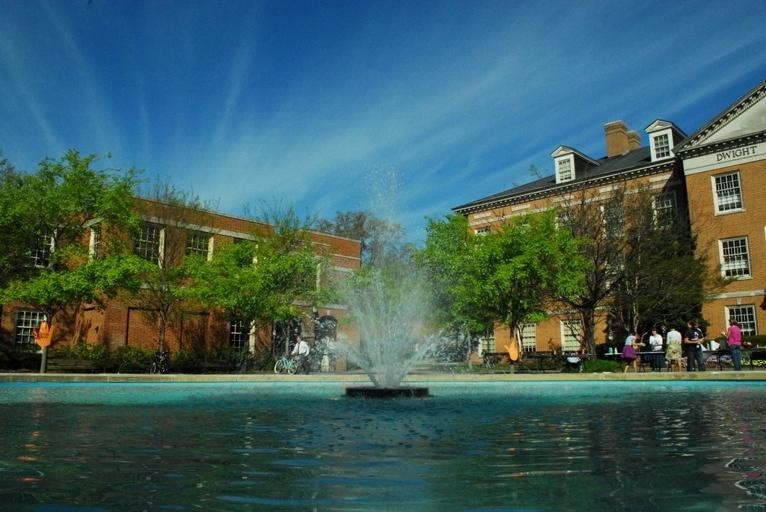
[151,351,171,374]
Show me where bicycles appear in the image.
[274,353,302,374]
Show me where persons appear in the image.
[720,318,742,371]
[684,319,706,371]
[648,327,664,371]
[290,335,311,373]
[664,324,682,371]
[694,320,704,338]
[621,332,646,373]
[658,324,670,371]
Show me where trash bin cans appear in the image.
[566,357,581,372]
[320,350,333,372]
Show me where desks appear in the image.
[604,349,761,373]
